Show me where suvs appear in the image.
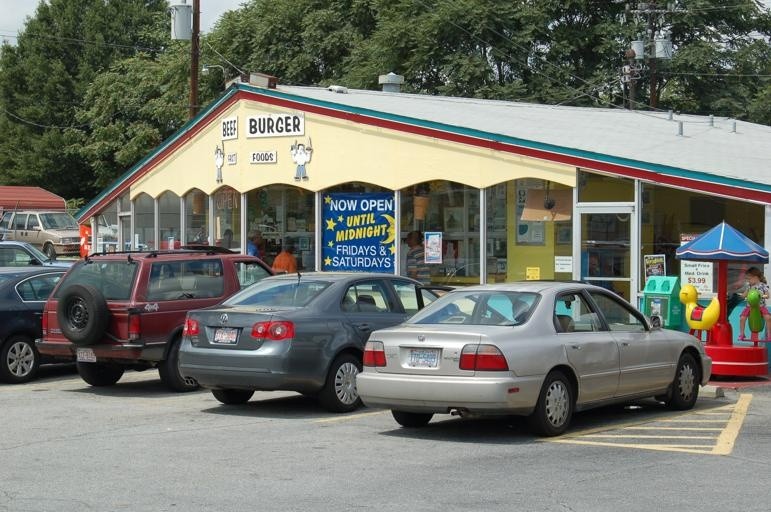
[32,247,276,393]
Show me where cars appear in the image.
[175,268,441,413]
[0,206,88,386]
[354,280,714,438]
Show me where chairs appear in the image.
[556,314,575,333]
[160,263,216,292]
[357,295,376,312]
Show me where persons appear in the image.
[219,227,239,249]
[733,265,771,340]
[213,147,225,182]
[290,142,313,181]
[270,236,297,274]
[247,229,263,258]
[588,247,625,298]
[403,230,437,288]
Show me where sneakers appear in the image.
[737,334,746,341]
[766,334,771,341]
[294,175,309,182]
